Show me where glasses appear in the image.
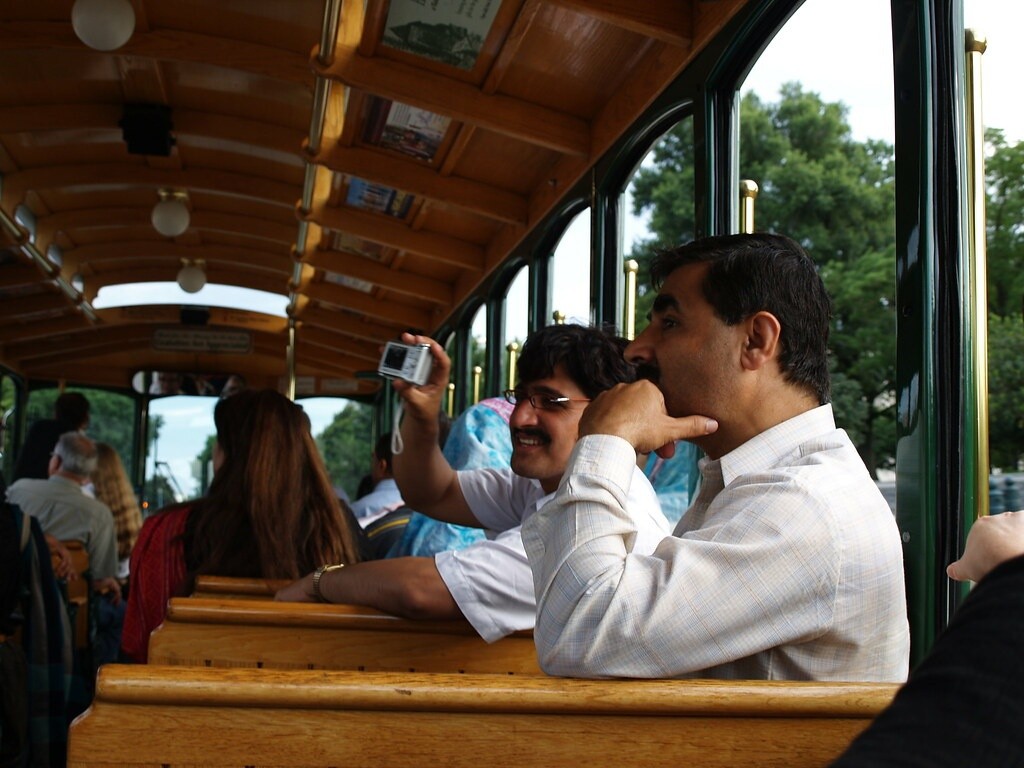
[504,390,591,411]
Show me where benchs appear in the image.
[58,539,907,768]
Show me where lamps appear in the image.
[71,0,207,293]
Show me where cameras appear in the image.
[378,343,434,386]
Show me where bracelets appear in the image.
[311,564,344,603]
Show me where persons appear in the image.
[832,511,1024,768]
[0,392,144,768]
[348,337,709,560]
[520,233,911,685]
[275,324,675,644]
[120,391,360,665]
